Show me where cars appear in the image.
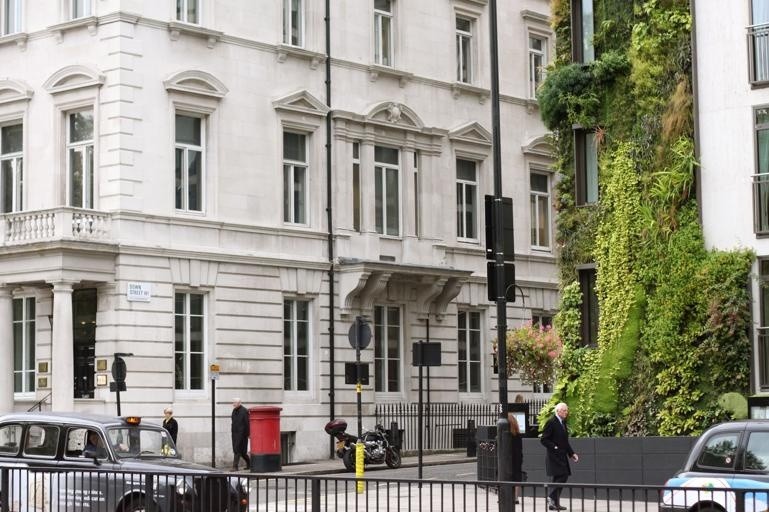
[656,418,768,511]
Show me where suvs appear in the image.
[0,412,249,511]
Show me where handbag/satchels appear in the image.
[522,472,528,482]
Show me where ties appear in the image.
[561,420,567,433]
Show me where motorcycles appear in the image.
[325,418,402,472]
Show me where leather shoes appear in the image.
[515,501,519,504]
[230,467,250,471]
[549,504,566,510]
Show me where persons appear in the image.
[230,398,249,472]
[515,394,524,403]
[78,430,129,458]
[508,413,522,505]
[540,403,579,510]
[160,408,178,453]
